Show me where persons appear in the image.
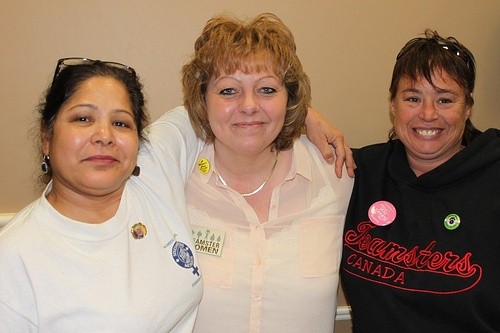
[182,13,355,333]
[339,29,500,333]
[0,57,356,333]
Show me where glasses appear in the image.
[50,57,136,94]
[397,38,470,71]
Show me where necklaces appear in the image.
[214,151,278,197]
